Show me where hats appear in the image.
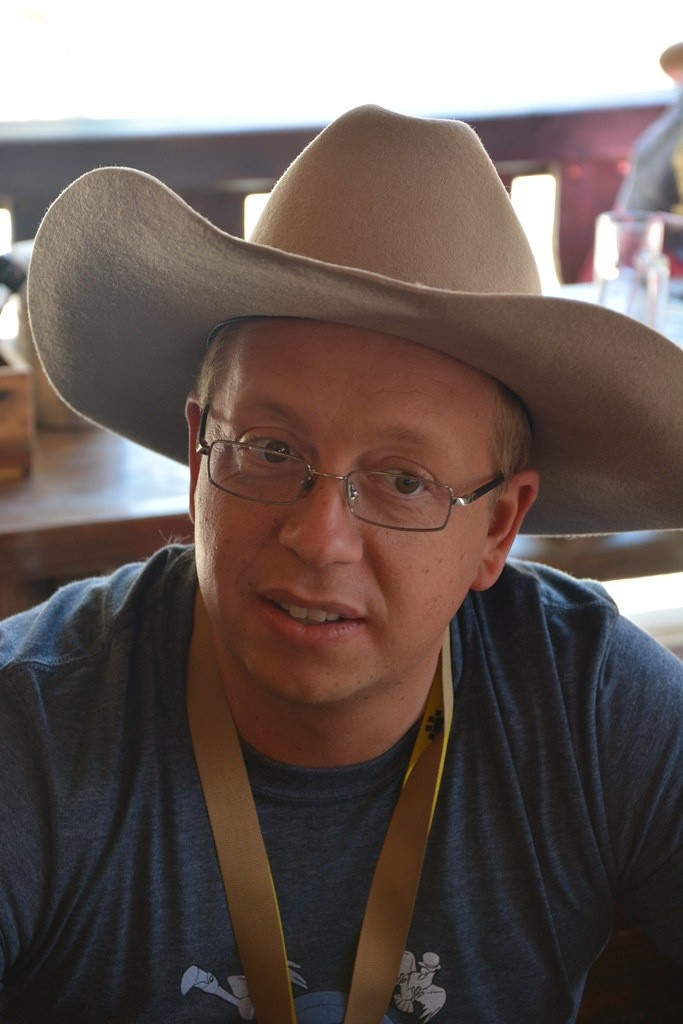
[26,104,683,536]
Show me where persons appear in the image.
[613,42,683,262]
[0,104,683,1024]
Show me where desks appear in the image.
[1,279,681,634]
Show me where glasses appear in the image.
[196,389,524,532]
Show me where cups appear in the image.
[592,211,672,337]
